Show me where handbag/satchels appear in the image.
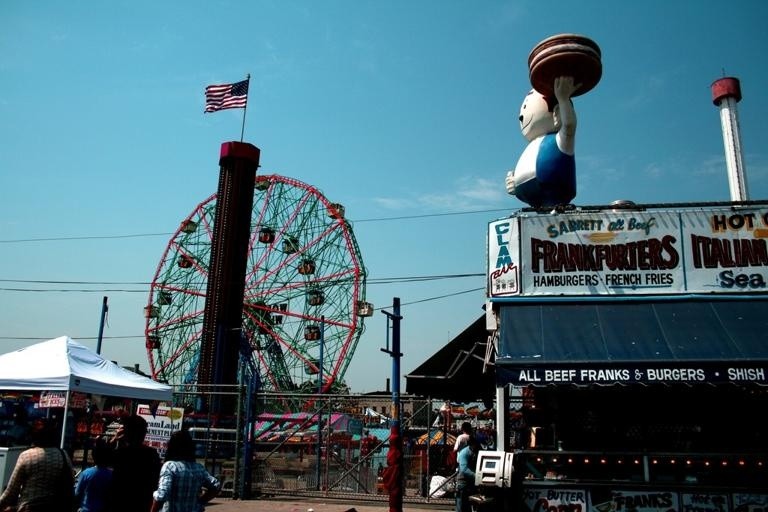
[49,463,80,512]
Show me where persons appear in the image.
[8,409,32,444]
[74,438,118,512]
[453,422,472,452]
[154,427,221,512]
[0,417,73,512]
[105,414,164,512]
[456,434,485,512]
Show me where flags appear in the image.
[203,78,249,113]
